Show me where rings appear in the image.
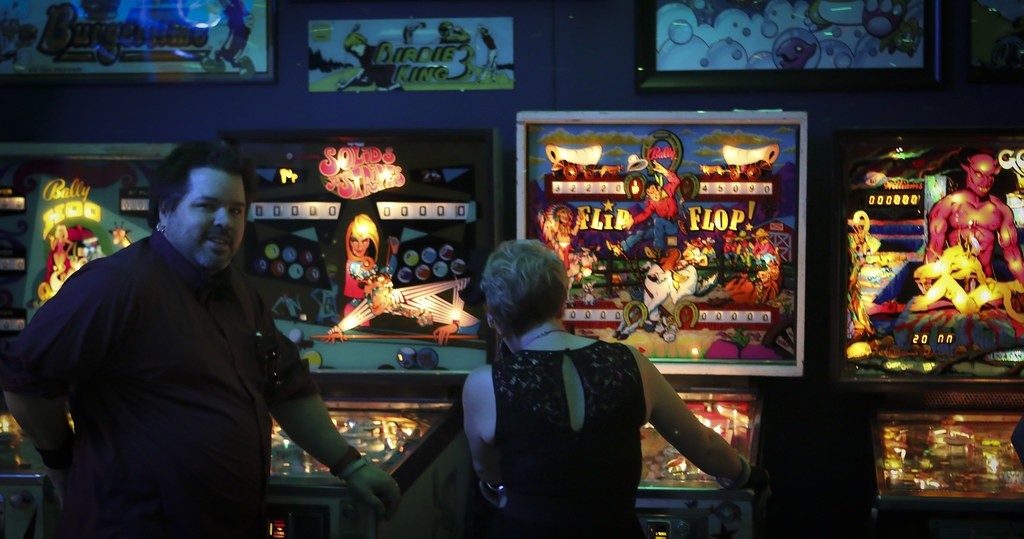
[394,481,399,488]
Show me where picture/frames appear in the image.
[0,0,277,84]
[517,106,807,377]
[632,0,941,90]
[218,126,501,344]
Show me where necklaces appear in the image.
[519,323,569,350]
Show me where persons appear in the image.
[4,143,405,539]
[462,240,772,539]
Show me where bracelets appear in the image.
[332,446,369,481]
[35,434,75,470]
[482,480,507,498]
[717,451,752,494]
